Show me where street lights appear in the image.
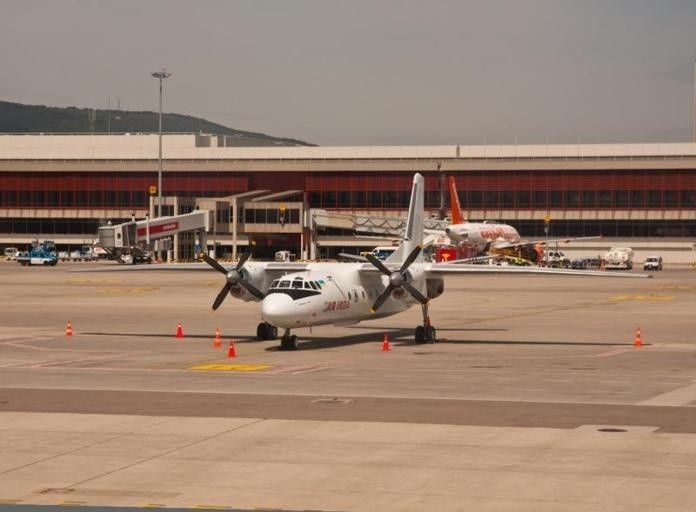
[152,68,172,250]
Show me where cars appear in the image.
[644,256,662,271]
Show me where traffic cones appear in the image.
[226,338,237,358]
[176,323,184,340]
[381,336,390,352]
[65,320,73,336]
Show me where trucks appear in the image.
[4,241,58,266]
